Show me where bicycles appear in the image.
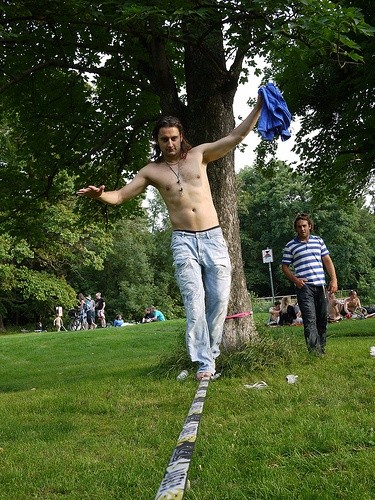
[70,312,85,332]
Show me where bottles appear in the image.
[177,368,189,380]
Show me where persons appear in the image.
[283,214,338,355]
[71,290,166,331]
[269,295,303,326]
[75,88,264,380]
[326,290,375,322]
[36,320,42,330]
[54,314,63,332]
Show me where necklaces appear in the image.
[161,157,184,192]
